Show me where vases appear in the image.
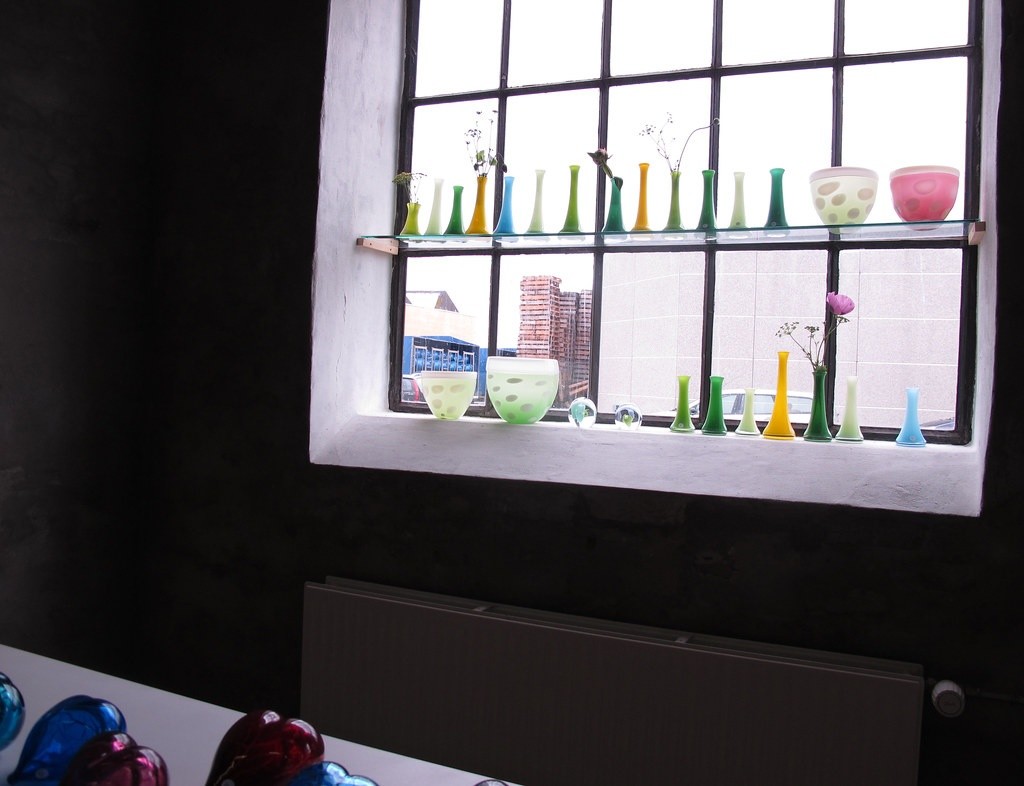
[803,370,832,442]
[557,166,585,241]
[669,376,695,433]
[727,172,751,239]
[809,167,878,234]
[763,168,790,238]
[631,163,652,238]
[421,371,477,419]
[601,179,626,238]
[399,203,421,235]
[493,177,519,243]
[890,165,959,231]
[735,389,761,436]
[444,186,465,234]
[662,171,687,240]
[835,376,864,441]
[465,177,490,234]
[896,387,926,447]
[424,179,445,235]
[701,375,727,436]
[486,356,559,424]
[524,169,549,240]
[762,352,795,440]
[695,171,721,239]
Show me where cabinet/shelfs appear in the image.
[357,216,986,451]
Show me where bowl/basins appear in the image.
[485,355,559,424]
[808,167,878,234]
[420,370,477,419]
[888,165,960,230]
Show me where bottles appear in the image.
[558,164,583,233]
[442,186,464,235]
[524,169,546,234]
[630,162,653,232]
[835,376,864,442]
[896,387,927,447]
[762,352,795,440]
[492,176,516,235]
[423,181,444,236]
[697,169,718,229]
[763,168,788,230]
[669,374,696,432]
[702,376,727,435]
[734,388,761,435]
[727,172,748,229]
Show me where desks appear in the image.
[0,645,527,786]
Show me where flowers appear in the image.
[775,292,855,370]
[587,148,623,191]
[465,110,507,177]
[639,111,720,171]
[393,172,427,203]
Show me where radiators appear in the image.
[301,576,924,786]
